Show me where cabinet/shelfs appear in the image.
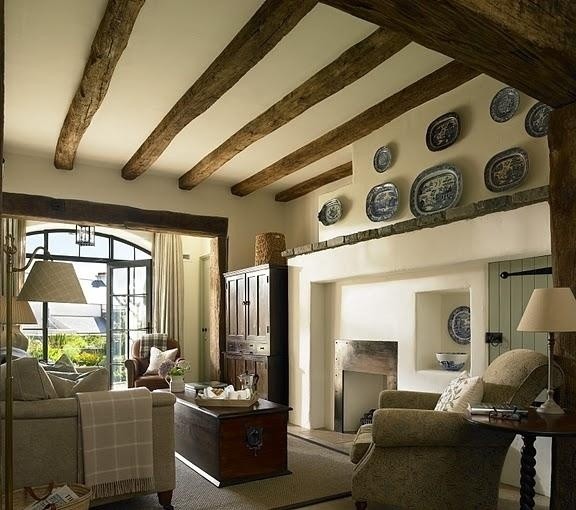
[221,351,289,423]
[223,263,288,356]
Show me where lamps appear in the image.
[75,223,96,247]
[0,295,39,351]
[517,286,576,416]
[3,234,86,509]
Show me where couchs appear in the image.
[348,349,564,509]
[0,356,176,508]
[124,333,181,391]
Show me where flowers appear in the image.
[158,357,191,379]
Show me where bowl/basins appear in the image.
[434,353,468,370]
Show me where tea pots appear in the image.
[237,370,259,393]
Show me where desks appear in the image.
[465,406,575,509]
[151,382,294,488]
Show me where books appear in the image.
[185,387,225,394]
[466,399,528,416]
[185,380,230,390]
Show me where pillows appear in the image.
[38,353,111,398]
[141,348,174,375]
[433,369,485,414]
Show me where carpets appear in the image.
[95,430,352,509]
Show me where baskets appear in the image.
[0,477,94,510]
[254,232,288,266]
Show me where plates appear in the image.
[447,304,472,347]
[316,87,552,227]
